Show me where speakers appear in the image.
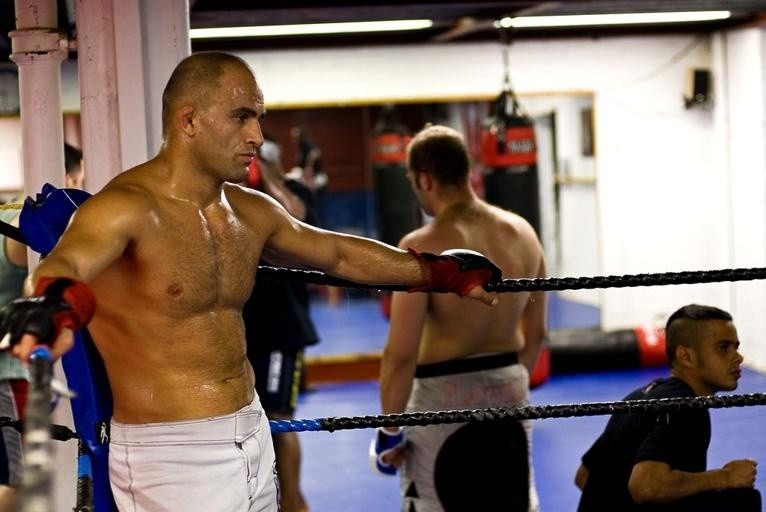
[684,68,711,107]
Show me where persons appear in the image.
[367,125,545,511]
[235,134,344,511]
[1,142,83,512]
[574,303,757,511]
[9,54,501,511]
